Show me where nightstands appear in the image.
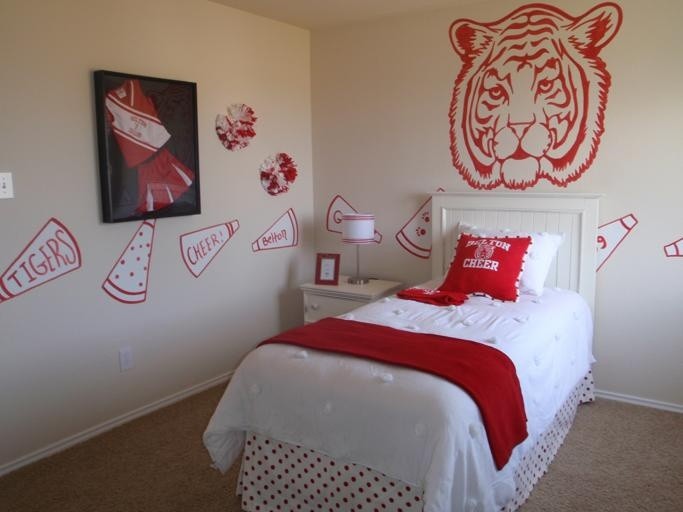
[298,274,403,327]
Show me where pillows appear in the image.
[432,221,564,304]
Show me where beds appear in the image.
[244,192,602,510]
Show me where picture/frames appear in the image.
[91,69,200,224]
[314,252,340,286]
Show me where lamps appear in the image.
[341,212,374,285]
